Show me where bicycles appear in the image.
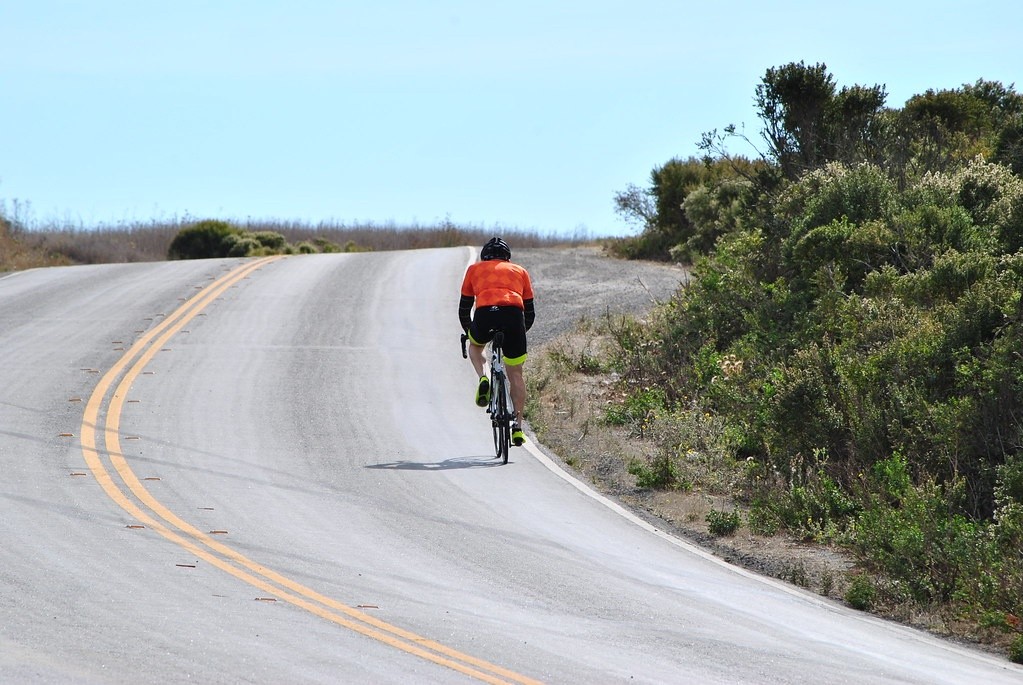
[461,331,522,464]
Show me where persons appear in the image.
[458,237,535,447]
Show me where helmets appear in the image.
[481,236,511,261]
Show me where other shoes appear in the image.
[475,375,490,407]
[511,424,526,445]
